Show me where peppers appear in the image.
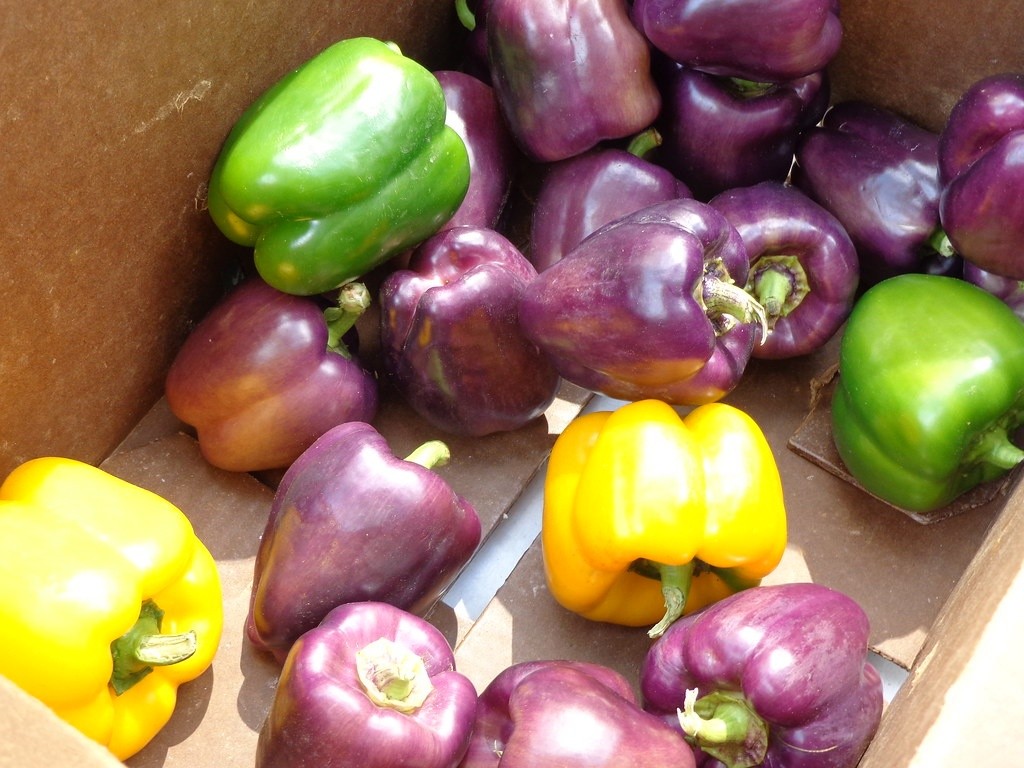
[0,0,1024,768]
